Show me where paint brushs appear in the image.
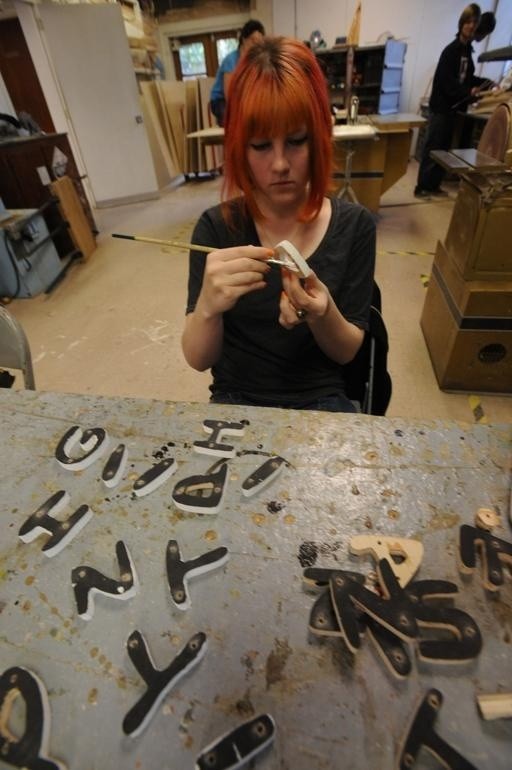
[112,232,294,268]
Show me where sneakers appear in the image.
[414,185,448,198]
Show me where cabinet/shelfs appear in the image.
[313,39,408,116]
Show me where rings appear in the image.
[294,308,309,319]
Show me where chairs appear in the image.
[1,307,36,391]
[334,280,382,415]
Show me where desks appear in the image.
[0,132,101,258]
[0,388,511,769]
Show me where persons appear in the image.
[210,19,265,128]
[415,3,499,200]
[181,35,376,414]
